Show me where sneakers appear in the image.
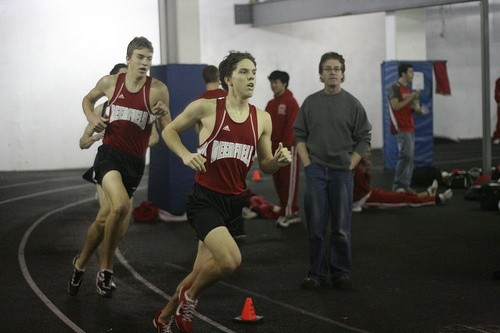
[437,189,453,204]
[95,264,114,299]
[152,311,173,333]
[68,255,86,296]
[276,216,301,227]
[427,179,438,197]
[175,286,198,333]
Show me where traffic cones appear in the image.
[234,298,264,324]
[251,169,261,181]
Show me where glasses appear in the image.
[322,65,343,73]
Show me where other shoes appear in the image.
[302,272,326,288]
[331,273,353,288]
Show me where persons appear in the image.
[151,50,294,333]
[292,52,373,293]
[67,37,500,297]
[387,63,424,193]
